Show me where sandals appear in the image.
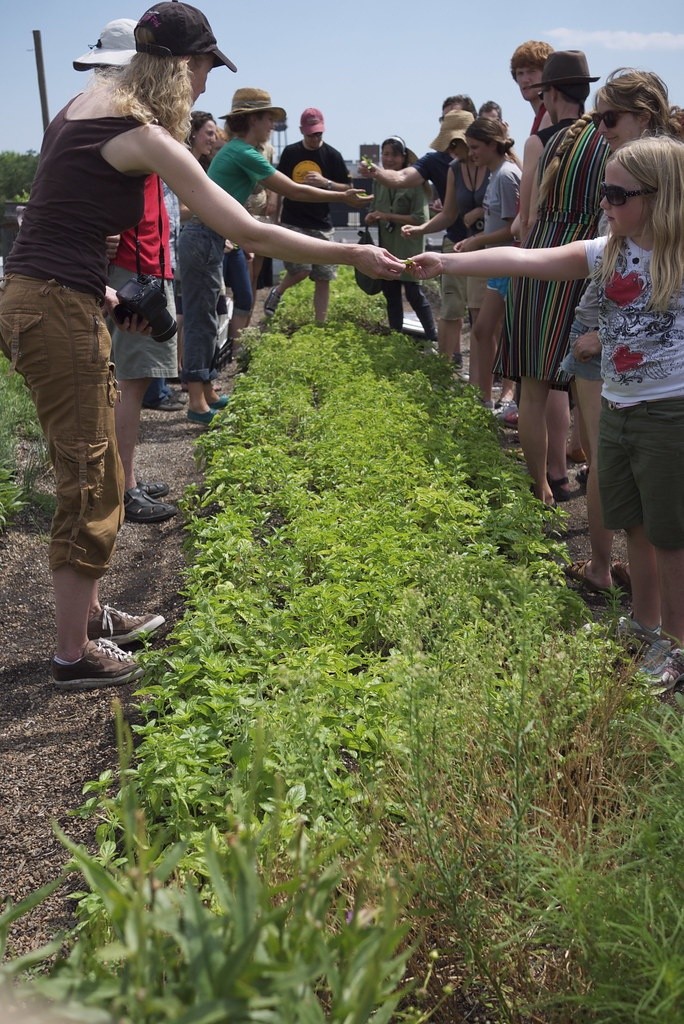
[123,487,177,522]
[135,481,169,498]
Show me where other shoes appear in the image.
[207,395,230,409]
[451,351,684,695]
[167,389,186,404]
[187,408,219,424]
[154,398,184,410]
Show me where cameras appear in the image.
[116,274,178,343]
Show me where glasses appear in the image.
[538,86,552,100]
[589,111,639,129]
[448,139,463,150]
[601,182,658,206]
[307,132,323,138]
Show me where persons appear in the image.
[265,108,352,324]
[0,0,407,689]
[72,18,176,525]
[562,68,683,598]
[247,144,275,292]
[406,136,683,690]
[141,111,227,411]
[364,135,437,342]
[490,113,611,510]
[360,40,601,503]
[178,88,374,426]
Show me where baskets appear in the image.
[213,338,235,372]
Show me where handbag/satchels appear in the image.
[354,226,388,295]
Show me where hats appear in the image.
[529,49,600,87]
[72,18,139,71]
[300,108,325,136]
[217,87,287,122]
[134,0,237,72]
[430,109,475,152]
[405,147,432,200]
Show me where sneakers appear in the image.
[51,637,145,688]
[88,605,166,645]
[263,286,281,316]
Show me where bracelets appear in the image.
[328,180,331,189]
